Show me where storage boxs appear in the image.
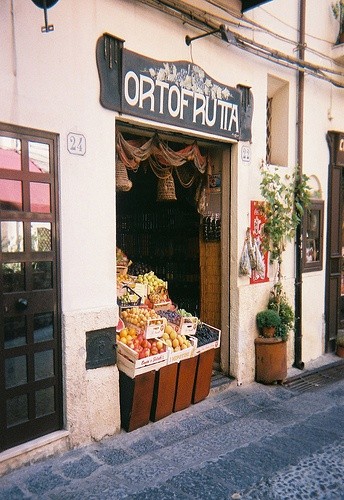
[115,266,221,379]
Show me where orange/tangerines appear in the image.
[116,328,137,345]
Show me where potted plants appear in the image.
[255,308,281,337]
[254,157,322,386]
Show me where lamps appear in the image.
[186,23,236,46]
[30,0,61,32]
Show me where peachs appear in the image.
[127,334,164,360]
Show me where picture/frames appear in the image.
[302,200,323,273]
